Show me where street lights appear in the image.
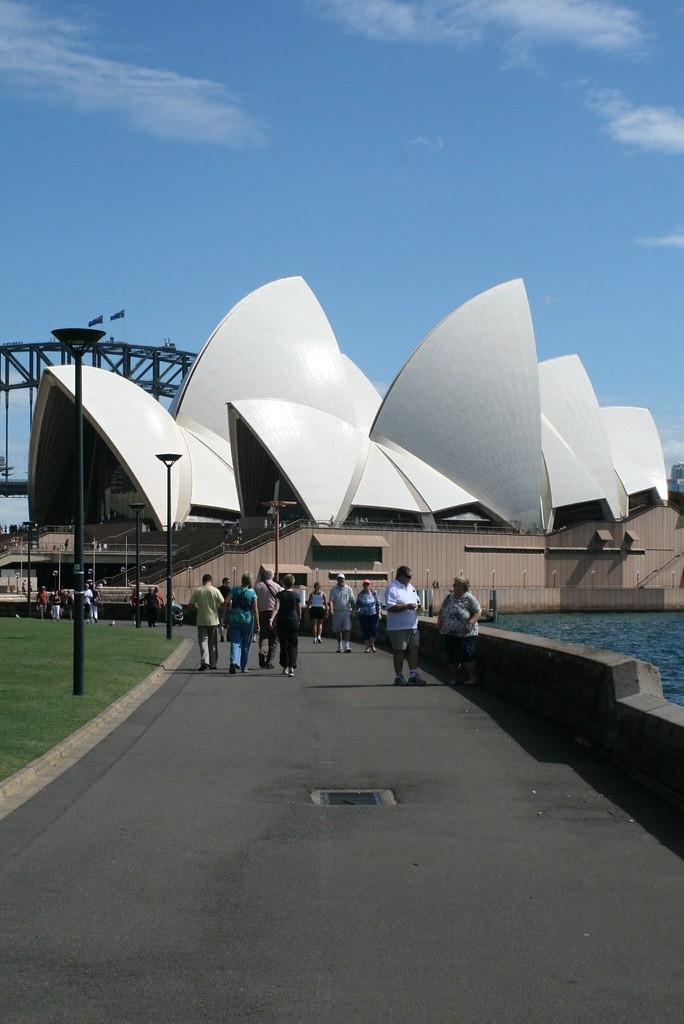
[121,567,125,586]
[88,568,93,587]
[155,454,184,634]
[50,328,111,697]
[15,573,19,595]
[128,503,147,629]
[21,521,38,618]
[53,571,58,592]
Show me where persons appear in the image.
[305,581,328,644]
[220,519,243,547]
[129,587,183,628]
[22,579,27,592]
[264,517,287,538]
[384,566,427,686]
[436,576,482,687]
[254,569,302,677]
[0,524,27,534]
[330,516,334,527]
[329,574,382,653]
[65,539,69,550]
[36,583,101,624]
[189,572,261,675]
[172,520,183,532]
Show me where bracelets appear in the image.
[378,612,381,614]
[269,619,273,621]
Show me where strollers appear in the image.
[172,604,186,627]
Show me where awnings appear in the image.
[312,533,390,548]
[596,530,614,541]
[626,530,640,541]
[260,564,313,574]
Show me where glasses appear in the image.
[404,573,411,579]
[453,584,456,586]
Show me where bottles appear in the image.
[417,601,423,616]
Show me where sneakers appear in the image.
[394,674,408,686]
[407,673,426,686]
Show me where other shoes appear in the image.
[221,635,224,642]
[259,654,266,668]
[242,670,249,673]
[450,677,475,684]
[314,637,322,643]
[337,647,351,653]
[364,643,376,653]
[198,664,217,671]
[230,662,236,674]
[283,670,294,676]
[266,665,274,669]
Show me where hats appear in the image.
[42,586,45,589]
[337,573,345,579]
[363,579,370,586]
